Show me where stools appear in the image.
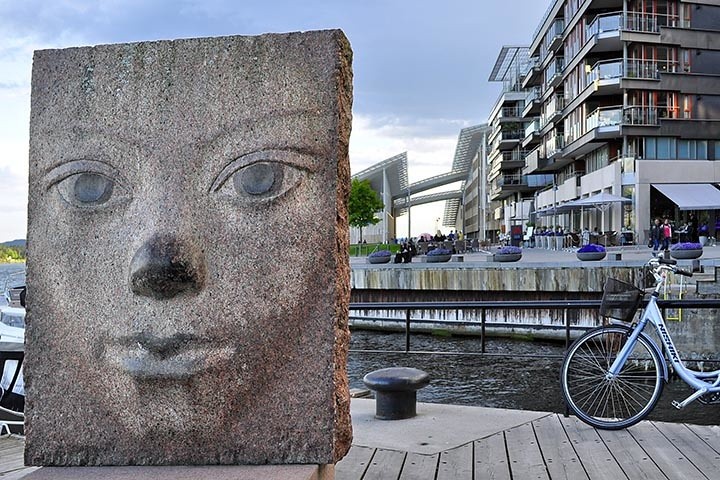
[364,367,430,420]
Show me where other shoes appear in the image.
[651,254,654,258]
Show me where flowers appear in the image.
[496,246,521,254]
[427,248,451,256]
[369,250,391,257]
[670,242,702,250]
[577,243,606,253]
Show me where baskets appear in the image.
[600,278,645,323]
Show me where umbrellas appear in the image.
[530,192,633,235]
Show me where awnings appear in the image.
[651,183,720,211]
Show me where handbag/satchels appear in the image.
[648,240,653,248]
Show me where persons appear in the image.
[26,105,334,466]
[394,225,599,264]
[649,213,716,259]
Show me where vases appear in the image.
[576,252,606,261]
[427,256,450,262]
[495,254,522,262]
[369,256,390,264]
[671,250,703,259]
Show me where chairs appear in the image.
[567,231,616,246]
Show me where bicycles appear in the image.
[559,257,720,430]
